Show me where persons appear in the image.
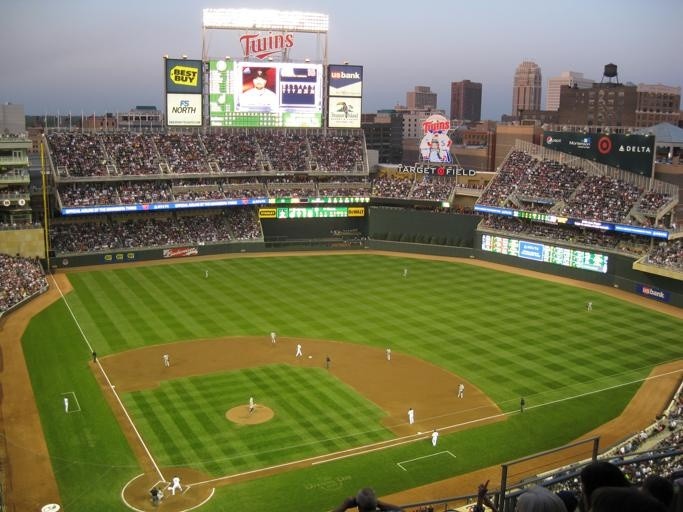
[471,389,682,509]
[161,350,170,369]
[44,130,482,253]
[407,406,415,426]
[324,353,331,370]
[1,127,49,315]
[171,474,182,495]
[204,268,210,279]
[156,487,165,501]
[385,344,392,363]
[585,296,594,313]
[62,394,70,415]
[149,485,158,504]
[456,381,466,398]
[270,329,277,345]
[327,485,407,512]
[431,427,439,447]
[399,266,408,278]
[471,142,683,277]
[239,70,278,109]
[248,393,256,415]
[91,348,98,364]
[293,343,304,360]
